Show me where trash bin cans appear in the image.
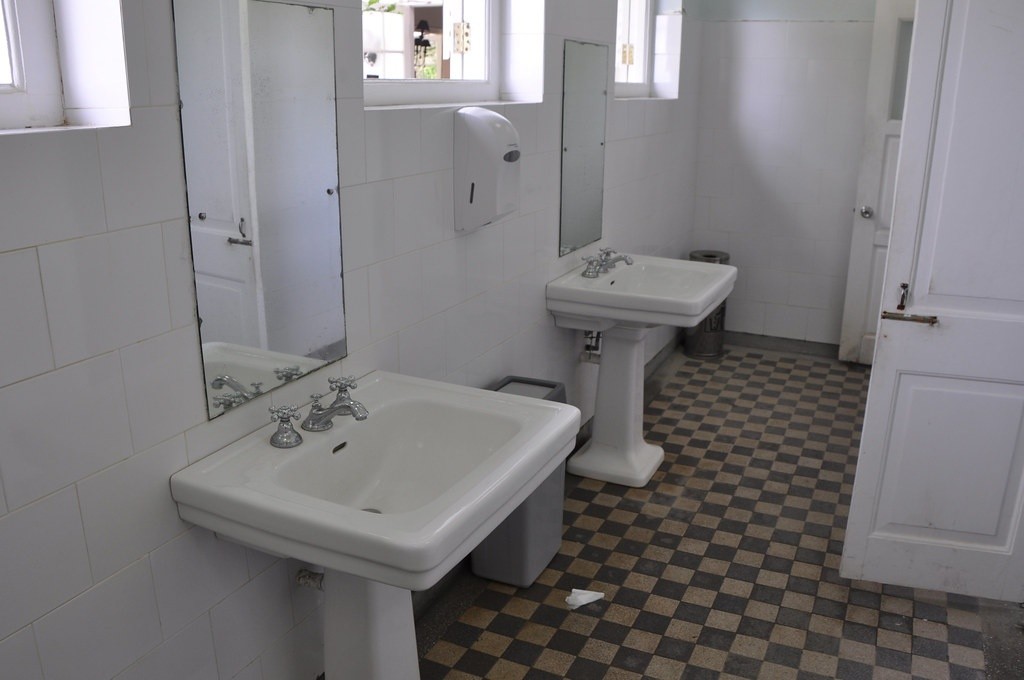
[470,376,567,588]
[683,249,730,359]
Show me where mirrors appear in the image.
[167,0,348,422]
[558,39,608,258]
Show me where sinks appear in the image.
[546,251,739,328]
[170,369,582,592]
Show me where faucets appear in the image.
[596,252,634,273]
[300,393,369,432]
[210,374,263,402]
